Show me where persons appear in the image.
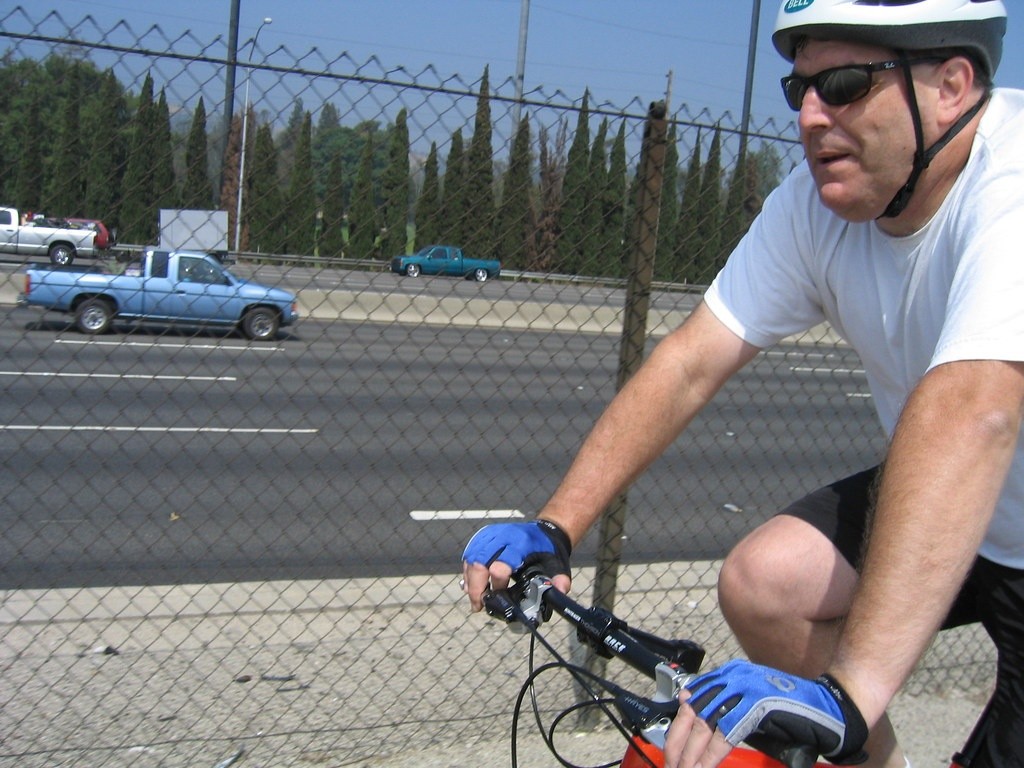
[462,0,1024,768]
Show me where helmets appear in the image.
[771,0,1007,83]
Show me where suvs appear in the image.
[40,217,118,251]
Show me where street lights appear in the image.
[236,16,273,263]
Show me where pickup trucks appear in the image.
[390,245,502,283]
[0,207,101,265]
[19,246,301,340]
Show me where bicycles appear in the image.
[458,566,850,768]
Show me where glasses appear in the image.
[780,55,947,111]
